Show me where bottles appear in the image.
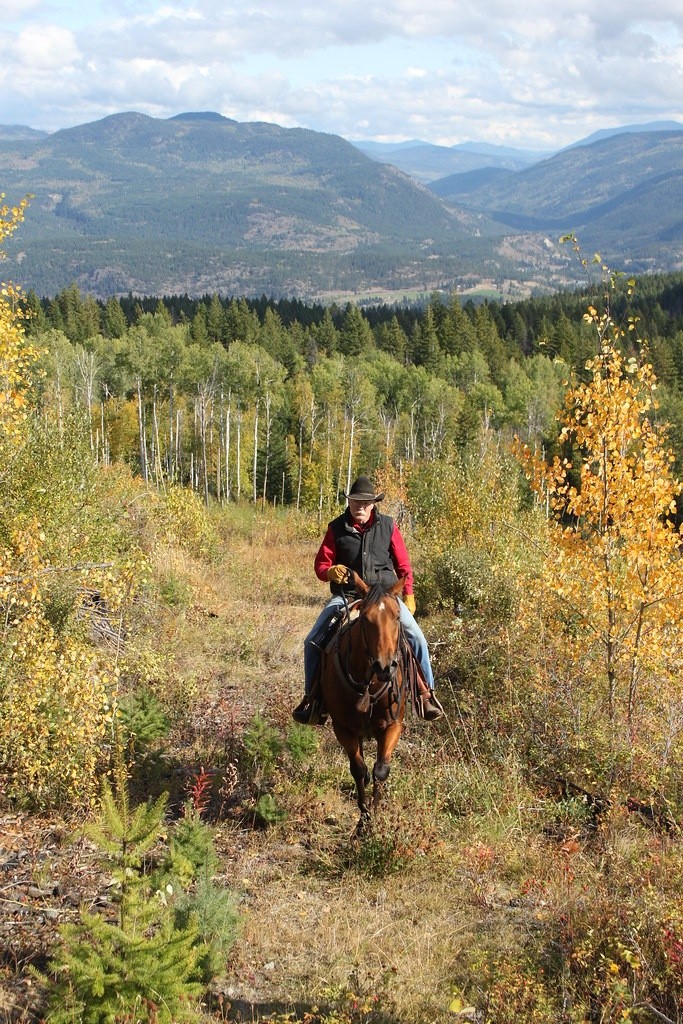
[312,610,343,649]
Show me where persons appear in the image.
[292,476,443,726]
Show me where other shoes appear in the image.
[292,703,328,726]
[417,699,441,719]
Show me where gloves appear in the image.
[327,564,351,584]
[402,594,416,616]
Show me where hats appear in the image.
[342,475,385,503]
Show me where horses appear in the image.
[321,571,411,848]
